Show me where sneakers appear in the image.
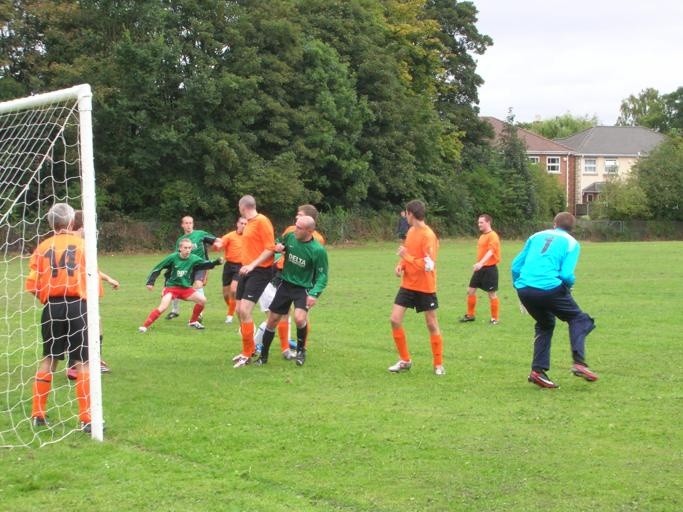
[80,420,107,435]
[66,360,111,380]
[32,415,53,428]
[434,365,445,376]
[459,314,476,323]
[528,370,558,389]
[388,359,413,373]
[188,311,205,329]
[281,338,307,367]
[490,318,499,325]
[572,363,599,382]
[138,326,147,333]
[225,315,233,324]
[232,343,268,369]
[164,311,180,320]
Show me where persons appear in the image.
[390,210,445,377]
[397,210,410,244]
[459,213,501,326]
[212,194,329,367]
[165,216,219,321]
[511,212,598,389]
[65,210,119,378]
[26,202,105,432]
[137,239,224,331]
